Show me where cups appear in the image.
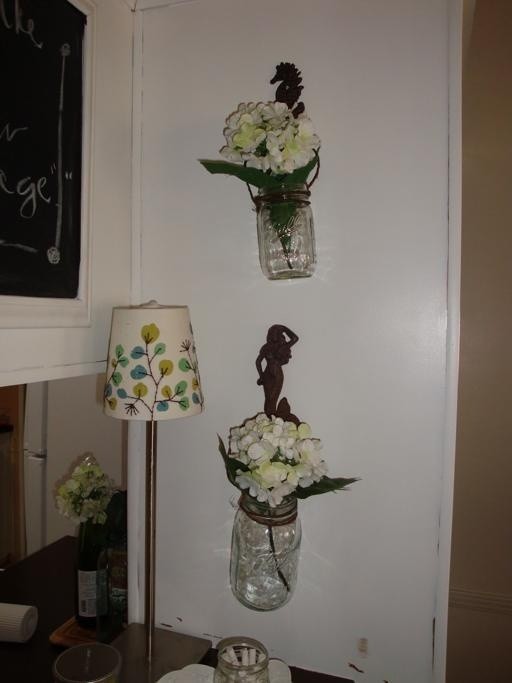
[53,642,122,683]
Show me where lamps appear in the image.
[103,300,212,683]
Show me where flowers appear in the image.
[216,413,362,593]
[54,456,119,525]
[196,102,321,269]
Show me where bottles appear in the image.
[97,533,128,641]
[214,635,269,683]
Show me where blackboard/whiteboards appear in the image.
[0,0,92,306]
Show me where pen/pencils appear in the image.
[220,642,268,681]
